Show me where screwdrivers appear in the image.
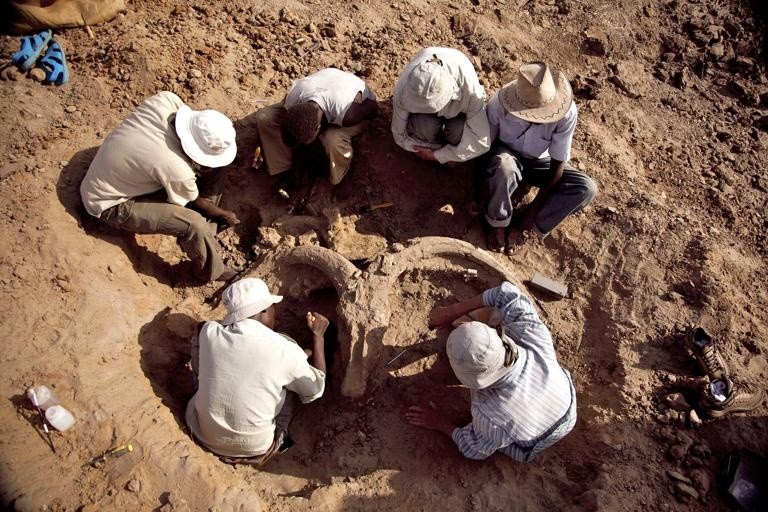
[387,319,441,366]
[81,444,134,469]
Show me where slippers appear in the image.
[487,231,505,254]
[506,228,527,255]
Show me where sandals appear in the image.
[12,29,52,72]
[40,39,69,85]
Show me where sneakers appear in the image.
[698,374,765,417]
[686,326,730,381]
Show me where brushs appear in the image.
[359,202,394,213]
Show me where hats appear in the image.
[497,61,574,124]
[446,320,517,392]
[400,61,455,114]
[221,278,286,326]
[174,105,238,168]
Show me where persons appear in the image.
[253,65,376,203]
[487,61,599,254]
[79,89,246,282]
[404,279,581,462]
[393,48,492,212]
[183,277,330,471]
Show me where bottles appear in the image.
[27,386,61,411]
[45,405,76,432]
